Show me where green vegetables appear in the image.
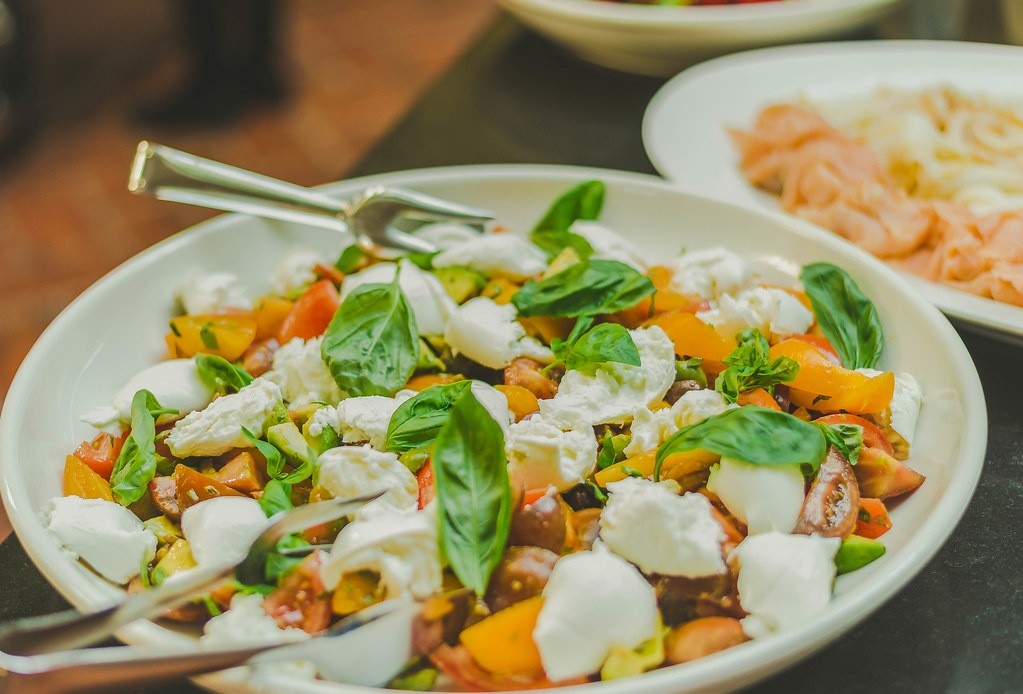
[106,179,884,611]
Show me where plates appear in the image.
[0,164,987,694]
[643,39,1023,345]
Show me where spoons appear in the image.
[0,586,477,694]
[0,490,386,657]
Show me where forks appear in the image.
[129,140,496,261]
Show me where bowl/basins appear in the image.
[496,0,904,77]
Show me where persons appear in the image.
[0,0,321,541]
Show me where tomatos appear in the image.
[63,272,939,694]
